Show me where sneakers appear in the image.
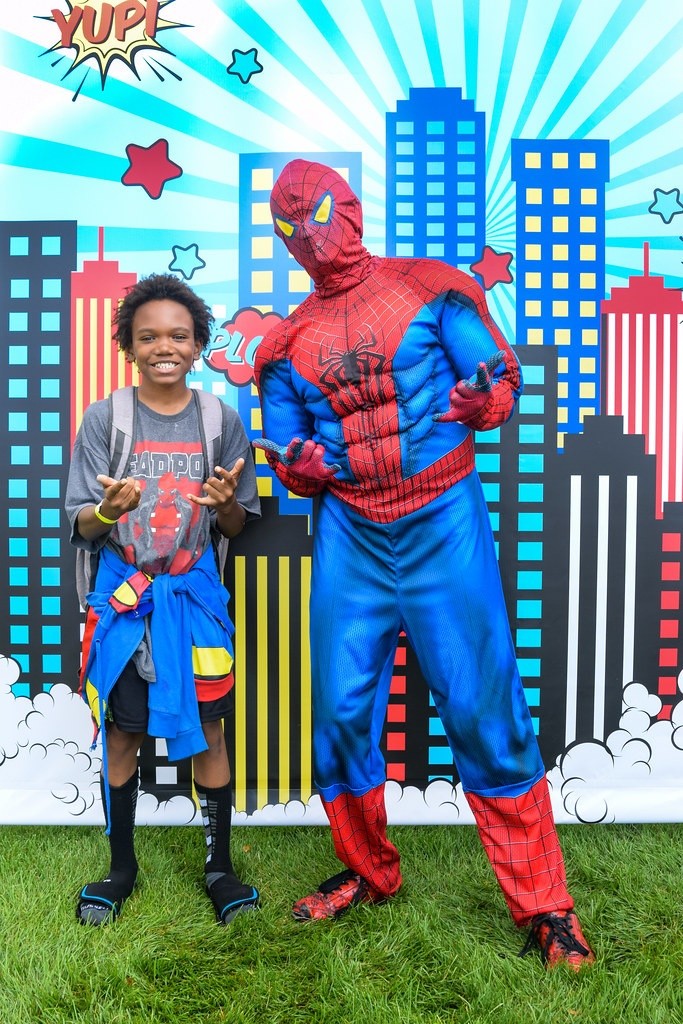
[518,909,597,974]
[291,869,384,923]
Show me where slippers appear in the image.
[206,876,259,925]
[76,882,125,927]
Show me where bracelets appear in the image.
[95,501,117,525]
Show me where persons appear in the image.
[251,159,594,975]
[66,272,262,926]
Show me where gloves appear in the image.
[432,350,506,423]
[252,438,342,480]
